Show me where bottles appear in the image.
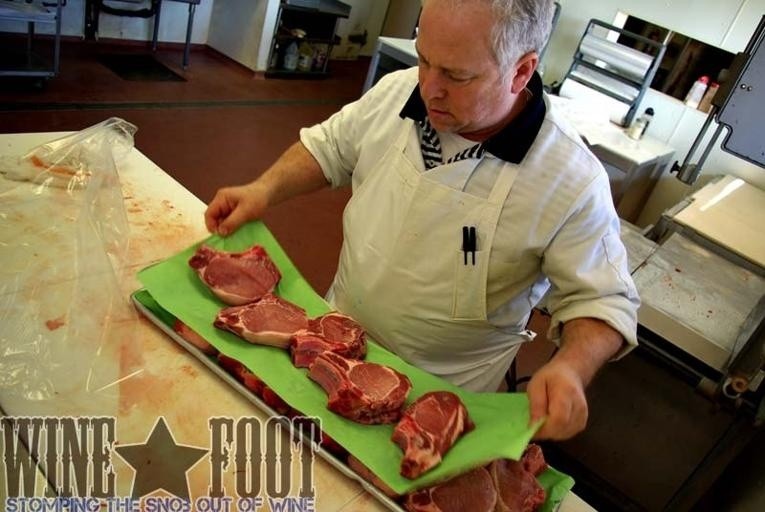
[685,76,720,113]
[629,107,654,140]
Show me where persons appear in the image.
[204,0,641,443]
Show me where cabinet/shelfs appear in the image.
[265,1,352,79]
[0,0,63,91]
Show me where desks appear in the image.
[361,36,677,224]
[0,127,599,511]
